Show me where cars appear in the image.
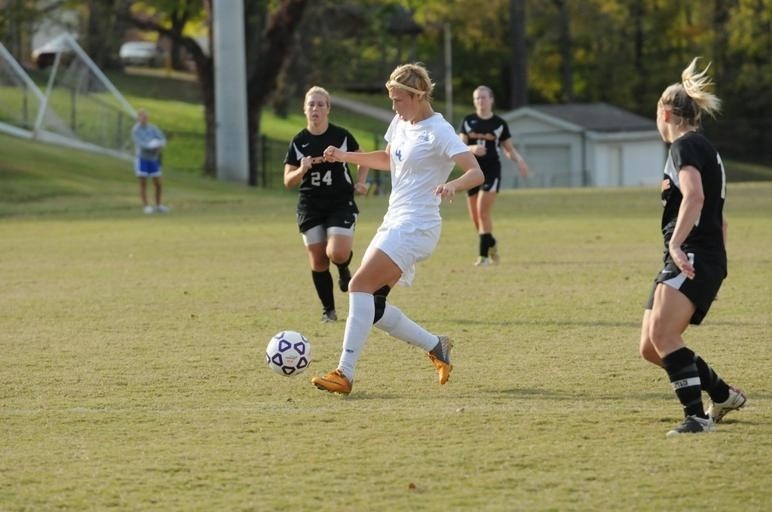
[119,39,159,67]
[32,33,81,70]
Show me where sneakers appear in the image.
[665,414,716,437]
[705,386,747,422]
[338,267,352,292]
[475,244,497,265]
[311,370,353,396]
[427,336,453,384]
[321,306,337,323]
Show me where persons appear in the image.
[130,109,170,214]
[311,62,485,397]
[283,85,370,322]
[459,85,527,266]
[639,55,746,437]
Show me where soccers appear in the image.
[266,330,310,376]
